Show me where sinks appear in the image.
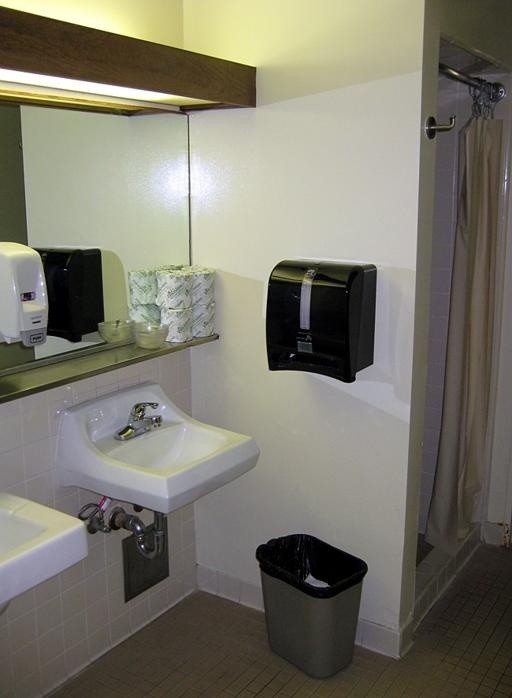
[0,493,88,604]
[55,382,260,515]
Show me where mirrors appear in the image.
[1,96,193,378]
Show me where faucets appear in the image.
[128,403,159,422]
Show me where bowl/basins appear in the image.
[131,320,171,349]
[96,318,133,345]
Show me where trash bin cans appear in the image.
[255,533,368,679]
